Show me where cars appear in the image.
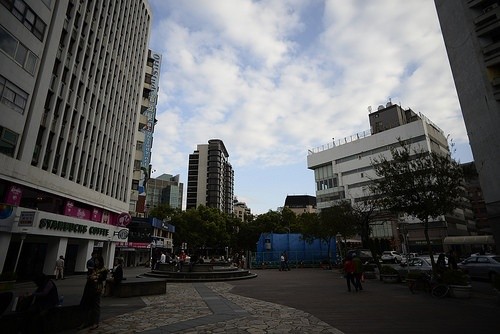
[402,253,448,270]
[457,255,500,283]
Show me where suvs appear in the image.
[381,251,402,263]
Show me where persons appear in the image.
[436,251,458,272]
[344,256,358,293]
[79,252,124,330]
[54,255,64,280]
[279,250,343,272]
[24,272,58,311]
[154,251,246,273]
[352,253,364,290]
